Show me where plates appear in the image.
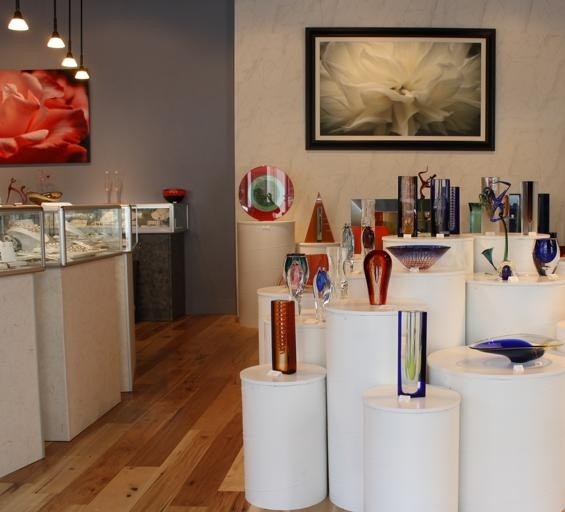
[465,332,565,363]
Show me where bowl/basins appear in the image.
[162,188,187,203]
[386,245,452,272]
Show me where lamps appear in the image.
[6,0,92,82]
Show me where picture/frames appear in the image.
[302,25,497,154]
